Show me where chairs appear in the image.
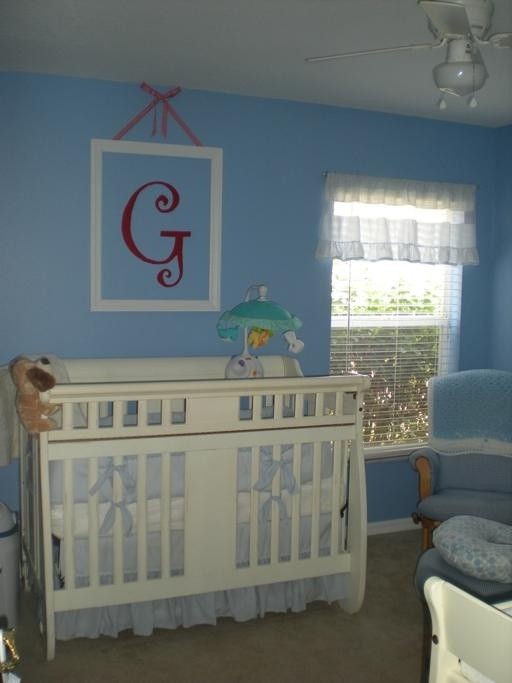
[409,369,512,551]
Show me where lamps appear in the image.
[431,40,490,110]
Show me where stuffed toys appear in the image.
[10,356,60,434]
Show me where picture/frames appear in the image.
[90,138,223,312]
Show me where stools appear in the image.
[414,547,512,683]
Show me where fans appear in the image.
[307,0,512,63]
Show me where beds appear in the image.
[19,358,371,661]
[423,575,512,683]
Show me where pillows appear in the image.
[433,514,512,582]
